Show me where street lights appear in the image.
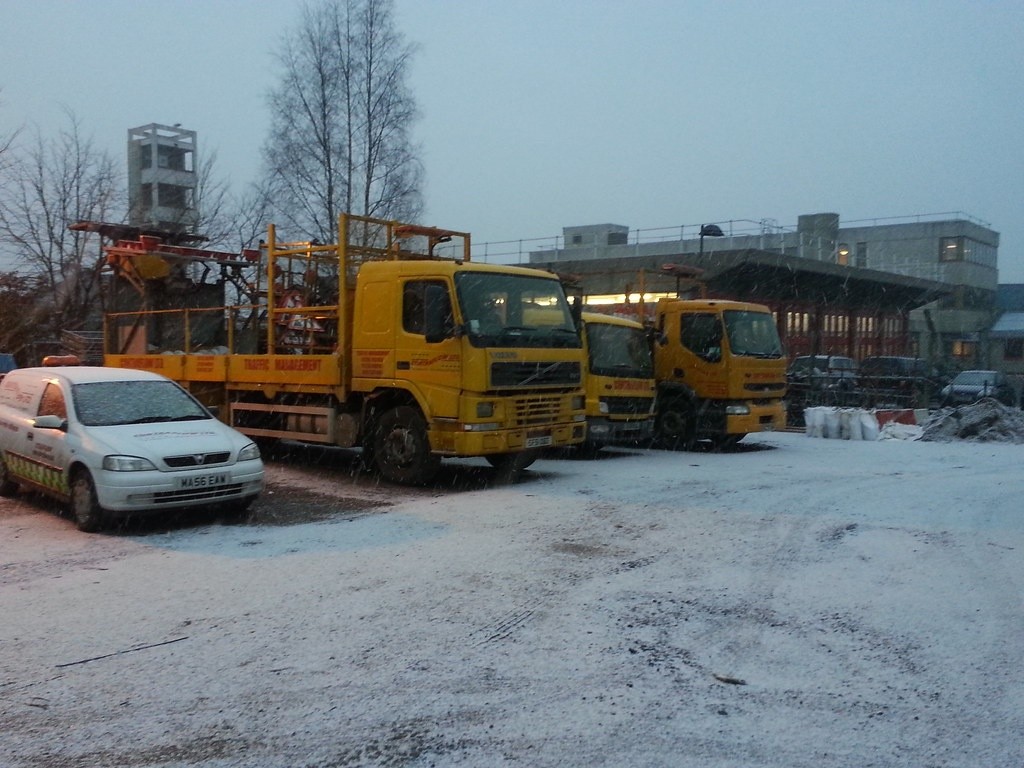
[693,222,725,265]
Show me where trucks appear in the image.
[100,211,587,483]
[505,307,658,460]
[563,264,789,455]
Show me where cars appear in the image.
[938,370,1018,407]
[0,355,265,534]
[857,354,941,396]
[791,357,858,377]
